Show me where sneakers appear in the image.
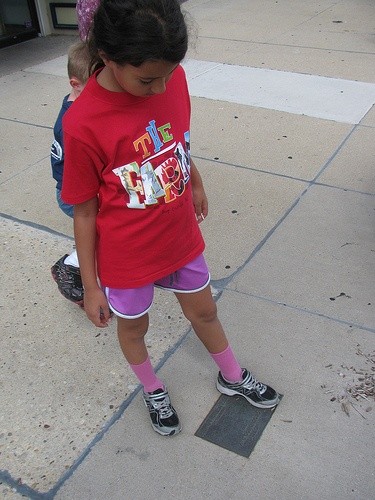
[143,389,182,437]
[50,254,84,301]
[216,368,278,409]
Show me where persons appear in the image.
[60,0,281,436]
[50,38,113,315]
